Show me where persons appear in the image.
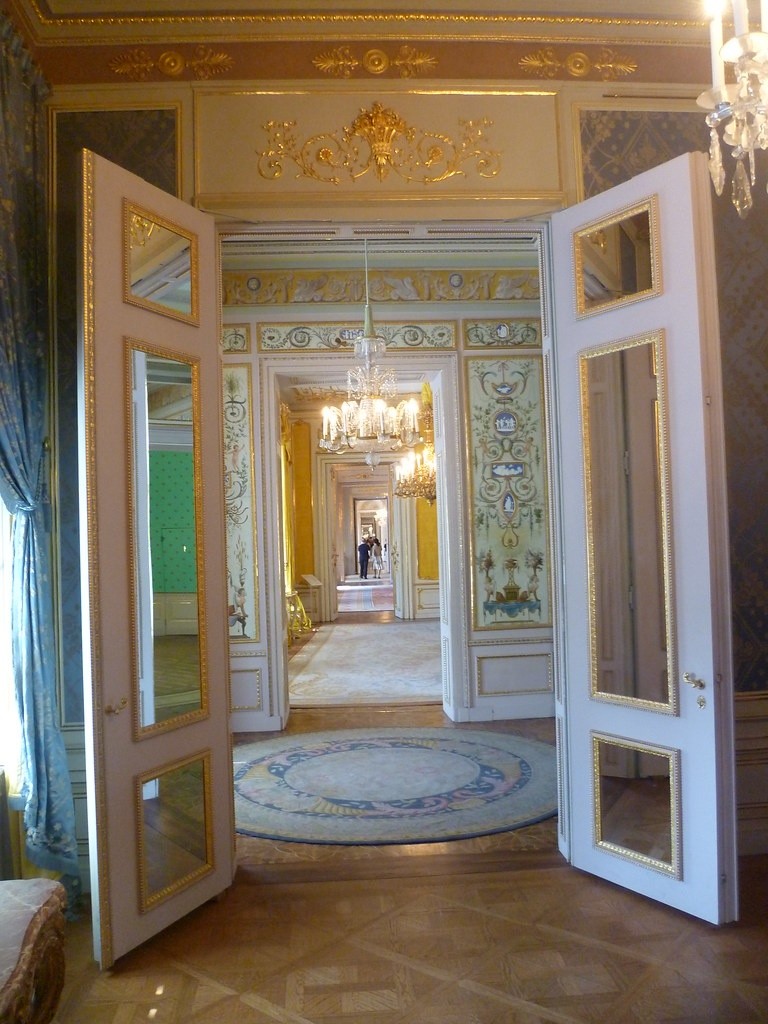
[371,538,383,579]
[358,538,371,579]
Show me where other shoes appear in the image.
[378,577,380,579]
[373,576,376,578]
[359,576,363,578]
[364,576,367,579]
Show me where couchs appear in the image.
[0,878,67,1024]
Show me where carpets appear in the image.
[233,726,558,845]
[337,586,394,612]
[287,624,442,707]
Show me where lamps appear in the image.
[318,237,425,471]
[394,380,440,508]
[695,0,768,218]
